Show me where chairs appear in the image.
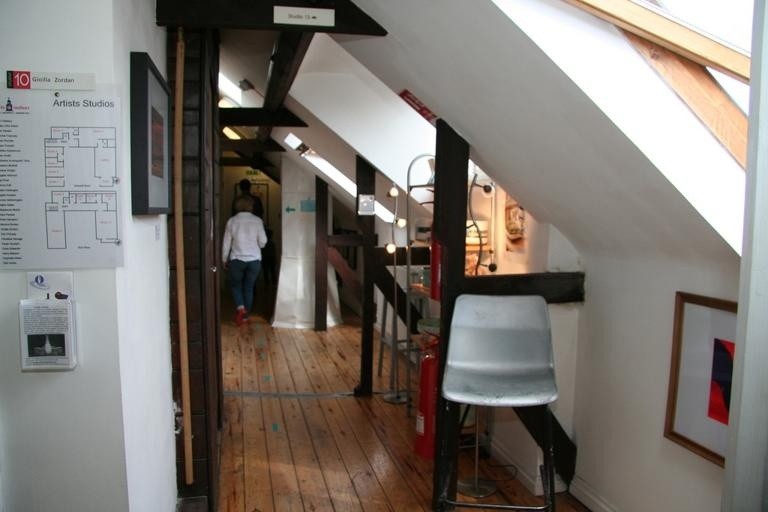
[436,292,559,512]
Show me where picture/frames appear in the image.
[663,291,738,469]
[130,51,172,215]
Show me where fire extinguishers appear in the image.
[413,329,440,458]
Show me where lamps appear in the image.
[466,174,497,276]
[218,73,303,151]
[300,147,395,223]
[385,183,409,403]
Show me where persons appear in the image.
[222,195,269,325]
[231,178,264,217]
